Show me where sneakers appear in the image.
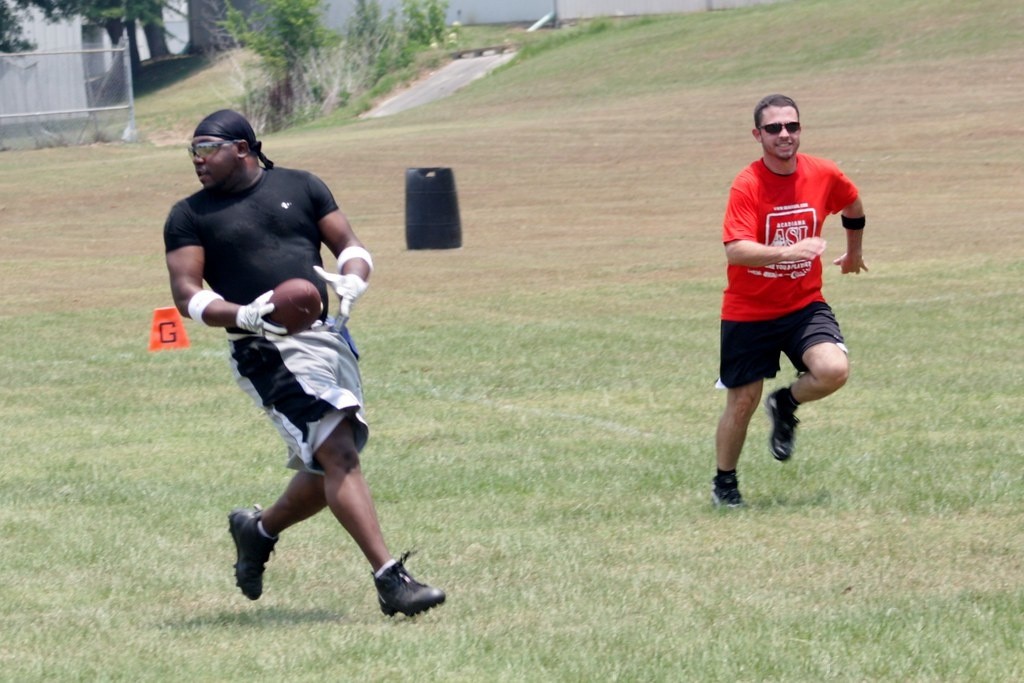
[372,551,446,617]
[228,503,278,601]
[767,386,799,462]
[712,471,744,510]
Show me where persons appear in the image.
[163,109,446,617]
[712,94,871,507]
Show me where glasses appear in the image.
[188,140,234,159]
[759,121,800,135]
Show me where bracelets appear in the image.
[841,214,866,229]
[337,246,373,273]
[187,289,224,324]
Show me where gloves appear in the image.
[236,290,288,343]
[314,266,368,331]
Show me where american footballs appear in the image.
[259,276,321,333]
